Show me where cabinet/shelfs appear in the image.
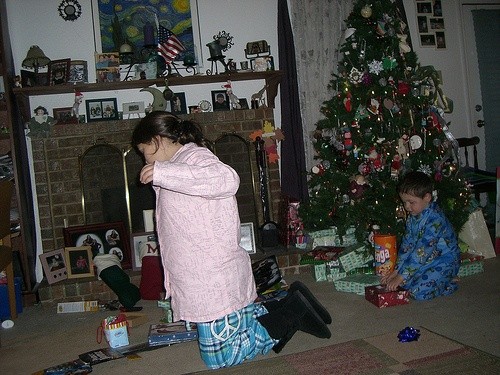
[0,0,33,295]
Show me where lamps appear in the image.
[21,45,51,85]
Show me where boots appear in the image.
[262,280,332,324]
[256,289,332,354]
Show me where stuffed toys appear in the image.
[71,92,84,118]
[221,80,242,110]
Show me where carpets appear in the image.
[182,326,500,375]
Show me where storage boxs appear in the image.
[307,228,486,308]
[56,301,99,315]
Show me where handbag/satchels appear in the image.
[97,318,131,349]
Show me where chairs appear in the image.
[0,183,20,321]
[435,136,497,207]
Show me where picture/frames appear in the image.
[85,97,119,123]
[61,221,132,271]
[211,90,231,111]
[53,107,80,125]
[39,244,95,285]
[90,0,203,71]
[239,222,258,254]
[416,0,449,51]
[129,232,162,273]
[170,92,187,114]
[48,58,88,85]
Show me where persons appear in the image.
[26,106,56,139]
[380,172,460,303]
[173,96,182,112]
[216,94,226,110]
[91,104,114,118]
[52,67,82,85]
[133,110,332,370]
[50,230,122,274]
[97,54,121,82]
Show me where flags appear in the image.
[157,22,184,64]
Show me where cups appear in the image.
[252,100,259,109]
[116,112,123,120]
[79,115,85,123]
[229,62,236,71]
[240,61,248,70]
